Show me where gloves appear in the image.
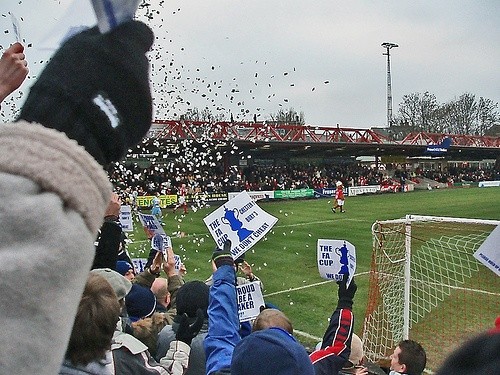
[17,20,153,170]
[212,238,235,269]
[176,308,205,346]
[337,272,358,308]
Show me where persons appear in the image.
[111,161,500,227]
[87,268,205,375]
[0,42,29,103]
[154,281,209,374]
[249,272,358,375]
[387,339,426,375]
[115,260,134,282]
[204,261,263,292]
[337,333,377,375]
[0,19,156,375]
[150,277,171,313]
[90,193,123,271]
[203,240,315,374]
[124,247,186,357]
[58,273,120,375]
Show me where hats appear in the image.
[340,332,364,370]
[90,268,133,302]
[175,281,210,320]
[124,284,157,321]
[115,260,134,277]
